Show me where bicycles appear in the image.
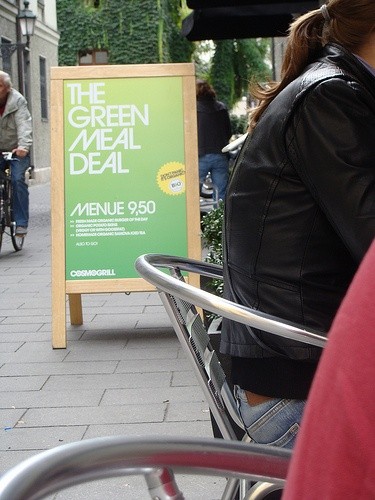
[0,150,33,254]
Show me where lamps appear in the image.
[0,1,37,60]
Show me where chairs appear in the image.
[0,253,329,500]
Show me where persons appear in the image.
[282,236,375,499]
[196,80,229,208]
[216,0,375,449]
[0,71,33,236]
